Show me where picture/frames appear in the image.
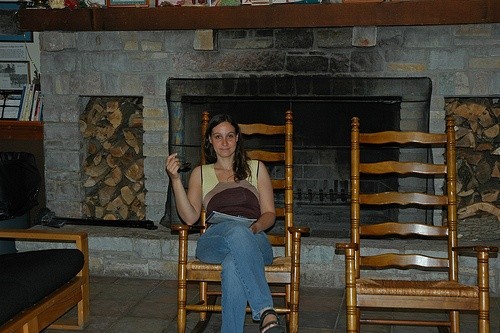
[0,58,32,90]
[0,0,34,42]
[105,0,150,7]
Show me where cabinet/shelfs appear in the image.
[0,119,47,228]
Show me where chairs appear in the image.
[172,108,312,332]
[334,112,499,332]
[0,226,91,332]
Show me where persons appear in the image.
[164,115,285,333]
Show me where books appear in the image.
[0,78,41,122]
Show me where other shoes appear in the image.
[259,310,283,333]
